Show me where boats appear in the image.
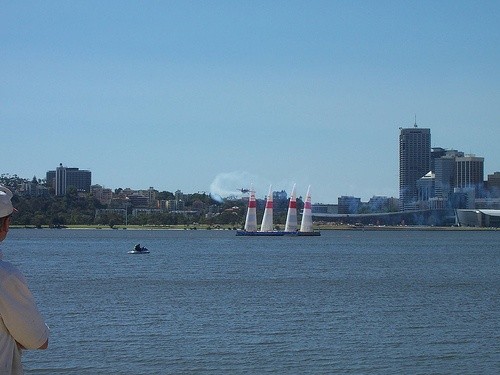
[127,246,151,254]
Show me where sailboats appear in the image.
[234,183,322,237]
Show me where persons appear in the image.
[0,186,50,375]
[135,244,141,252]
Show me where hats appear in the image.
[0,186,20,218]
